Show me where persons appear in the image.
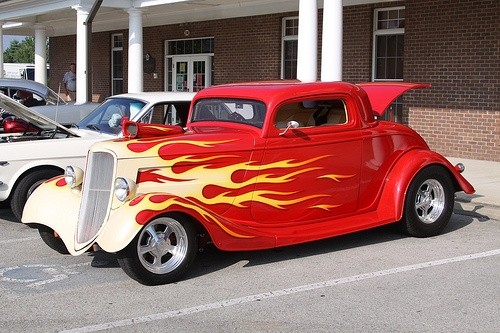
[62,63,76,101]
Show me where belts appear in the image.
[68,89,76,92]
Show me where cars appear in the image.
[21,81,476,287]
[1,91,253,228]
[0,78,101,128]
